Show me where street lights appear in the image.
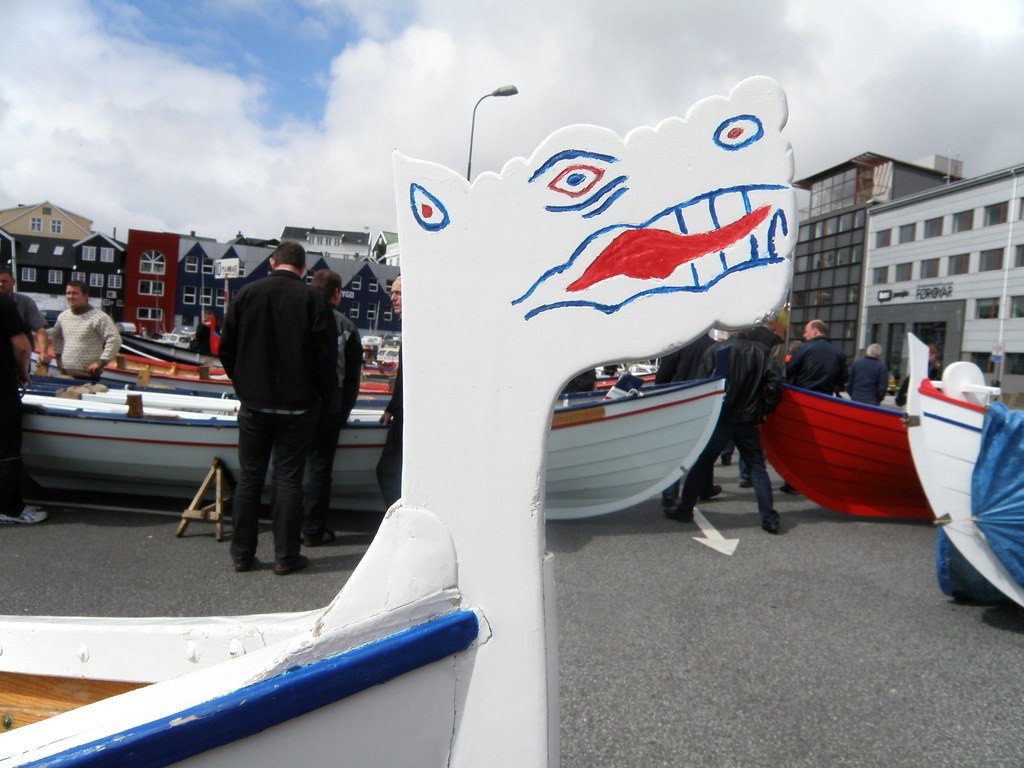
[466,84,519,182]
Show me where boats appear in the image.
[16,375,725,519]
[1,74,799,768]
[119,332,223,368]
[758,381,934,519]
[27,331,393,408]
[905,331,1024,608]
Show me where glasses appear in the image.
[388,291,401,297]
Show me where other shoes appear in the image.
[661,496,674,507]
[664,507,694,523]
[762,517,780,534]
[739,478,753,488]
[779,486,797,495]
[700,485,722,500]
[236,560,255,571]
[0,503,48,525]
[721,451,732,465]
[304,529,335,547]
[273,555,308,575]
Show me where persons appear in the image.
[928,343,941,391]
[562,365,618,393]
[655,324,788,534]
[45,280,122,385]
[846,344,889,407]
[376,270,403,547]
[196,310,220,355]
[0,266,51,526]
[779,320,849,496]
[216,243,340,575]
[301,269,362,547]
